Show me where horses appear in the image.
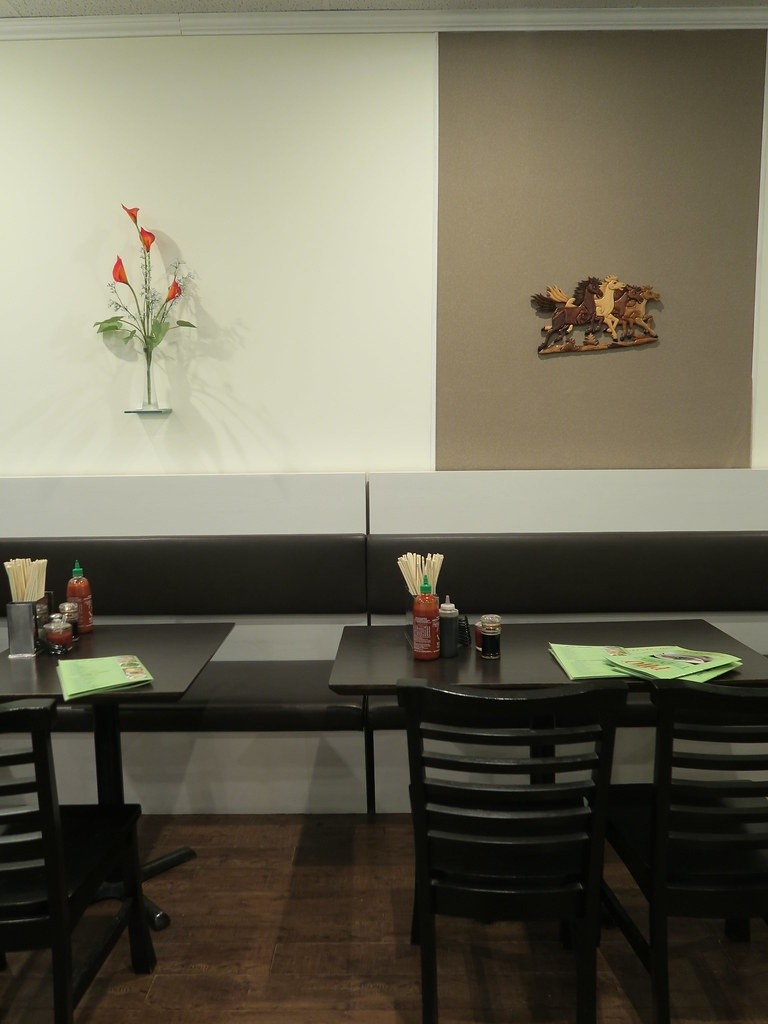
[610,284,644,341]
[541,274,626,345]
[625,284,660,337]
[530,276,605,353]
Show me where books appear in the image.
[548,640,744,684]
[55,653,154,702]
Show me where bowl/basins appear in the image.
[42,613,74,655]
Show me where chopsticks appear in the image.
[397,551,444,595]
[3,559,48,603]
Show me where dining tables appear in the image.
[332,614,754,940]
[1,619,238,971]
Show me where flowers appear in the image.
[93,205,198,363]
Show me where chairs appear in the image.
[0,706,157,1023]
[586,675,768,1024]
[383,671,632,1024]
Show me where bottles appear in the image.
[413,573,440,660]
[438,594,459,658]
[480,614,502,659]
[67,558,94,635]
[59,602,80,642]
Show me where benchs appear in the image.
[0,529,767,807]
